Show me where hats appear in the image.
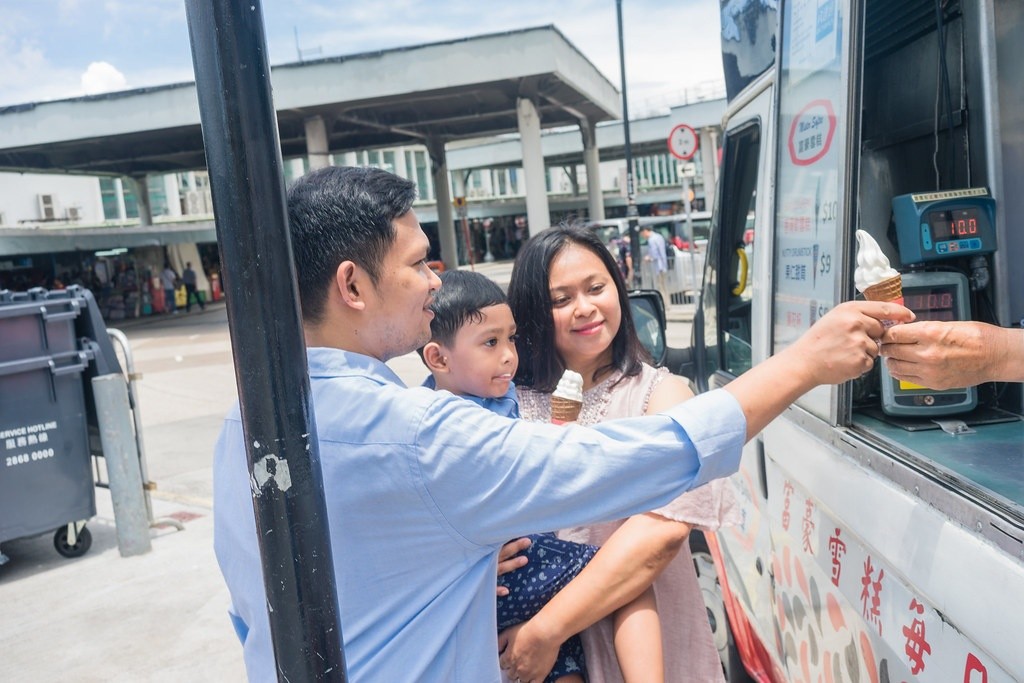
[639,223,654,232]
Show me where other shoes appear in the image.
[186,309,191,312]
[165,309,178,315]
[200,305,206,309]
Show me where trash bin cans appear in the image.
[1,285,131,574]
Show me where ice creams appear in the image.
[854,229,905,328]
[550,369,584,425]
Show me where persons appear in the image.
[880,320,1024,391]
[181,262,205,313]
[158,263,179,315]
[211,166,915,683]
[608,229,633,289]
[639,226,668,310]
